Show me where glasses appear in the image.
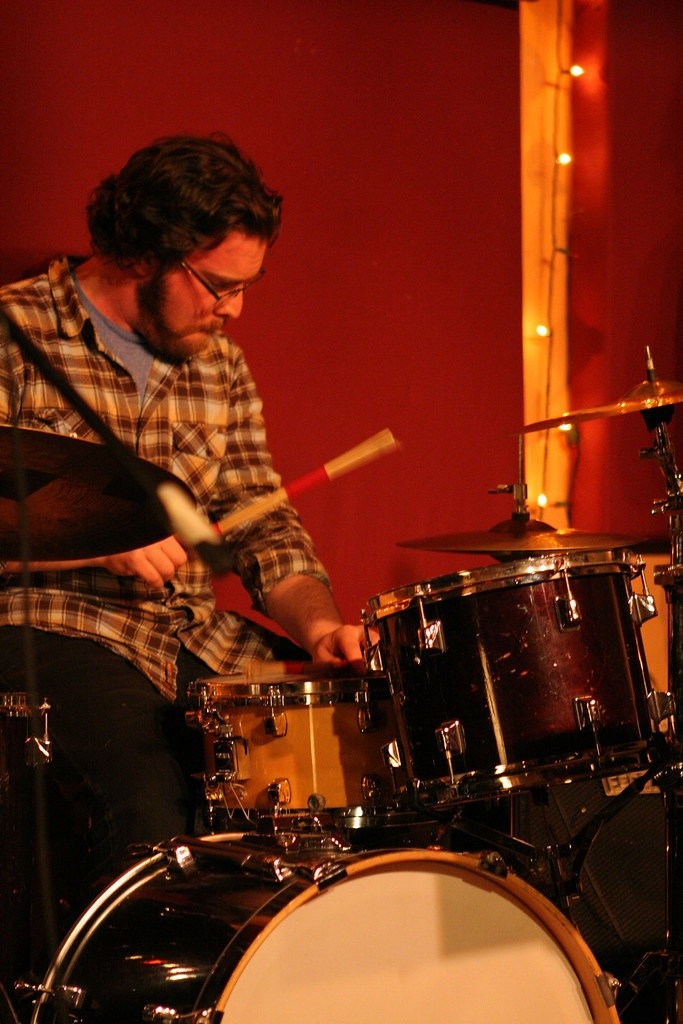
[176,256,264,310]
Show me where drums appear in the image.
[25,828,625,1022]
[364,546,674,816]
[190,669,400,819]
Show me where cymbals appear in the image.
[514,378,683,440]
[0,422,196,564]
[393,517,652,556]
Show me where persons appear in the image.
[0,131,380,942]
